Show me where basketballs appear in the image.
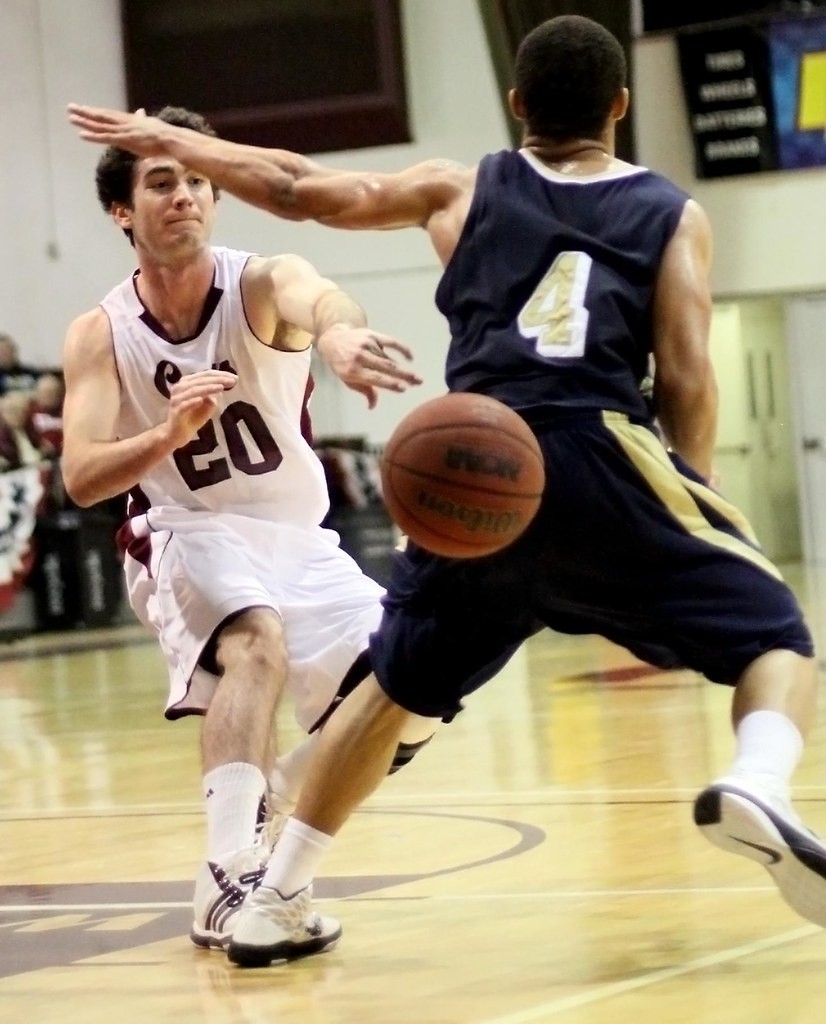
[381,392,542,559]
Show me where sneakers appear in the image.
[190,835,268,951]
[693,774,826,928]
[255,788,298,867]
[227,877,343,967]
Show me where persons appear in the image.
[62,105,424,946]
[0,336,127,624]
[63,14,826,969]
